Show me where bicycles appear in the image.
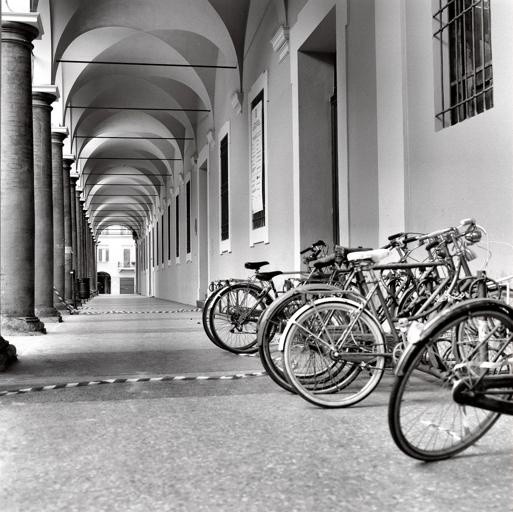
[256,218,513,410]
[387,297,513,462]
